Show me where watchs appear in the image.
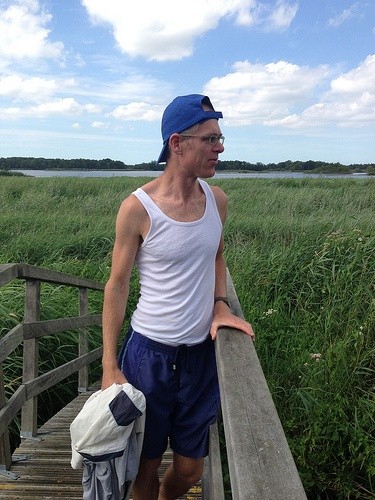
[213,297,230,307]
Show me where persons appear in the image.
[101,94,257,500]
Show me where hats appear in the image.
[156,94,224,165]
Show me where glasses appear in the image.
[179,133,225,145]
